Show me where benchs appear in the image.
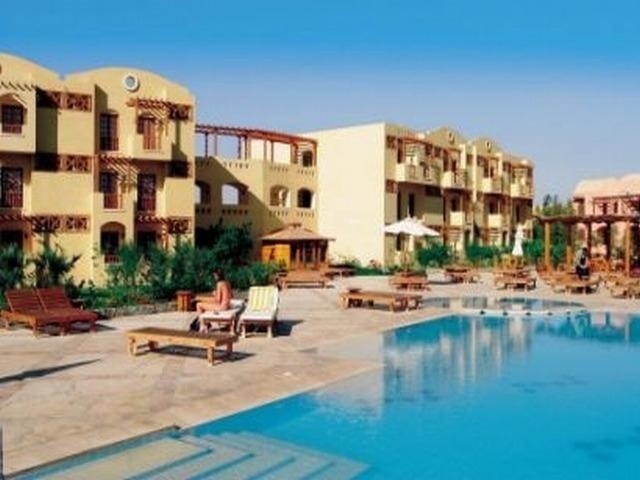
[126,326,239,367]
[280,277,329,289]
[339,289,421,314]
[387,276,430,290]
[494,277,536,291]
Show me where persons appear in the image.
[196,268,233,334]
[575,248,589,280]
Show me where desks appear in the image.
[399,272,420,290]
[502,269,529,291]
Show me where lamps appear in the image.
[158,100,183,121]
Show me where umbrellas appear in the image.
[383,215,441,277]
[512,221,524,279]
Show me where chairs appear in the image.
[198,283,280,339]
[0,287,100,339]
